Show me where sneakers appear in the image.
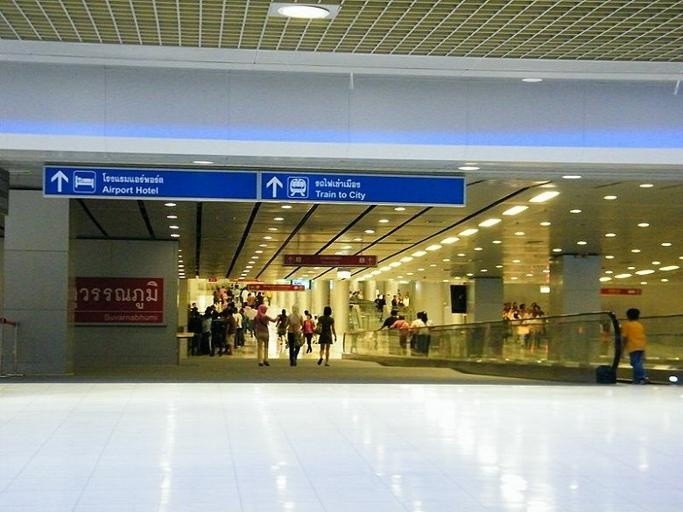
[259,360,270,366]
[326,364,330,367]
[317,359,322,364]
[290,360,295,365]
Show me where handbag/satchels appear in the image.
[316,323,323,333]
[297,332,306,344]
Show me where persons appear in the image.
[187,284,337,366]
[349,290,363,311]
[618,309,650,385]
[502,302,549,354]
[600,323,613,364]
[374,294,410,354]
[409,311,432,356]
